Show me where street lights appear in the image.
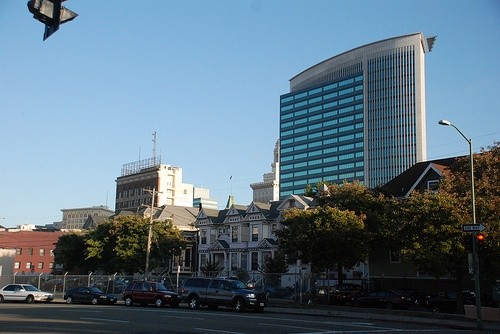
[437,119,483,328]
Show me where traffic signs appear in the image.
[462,224,485,231]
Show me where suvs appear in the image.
[122,280,180,308]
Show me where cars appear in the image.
[16,278,125,295]
[292,284,488,313]
[0,284,54,304]
[64,287,117,305]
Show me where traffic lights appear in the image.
[477,234,484,245]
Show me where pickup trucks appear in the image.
[181,277,264,312]
